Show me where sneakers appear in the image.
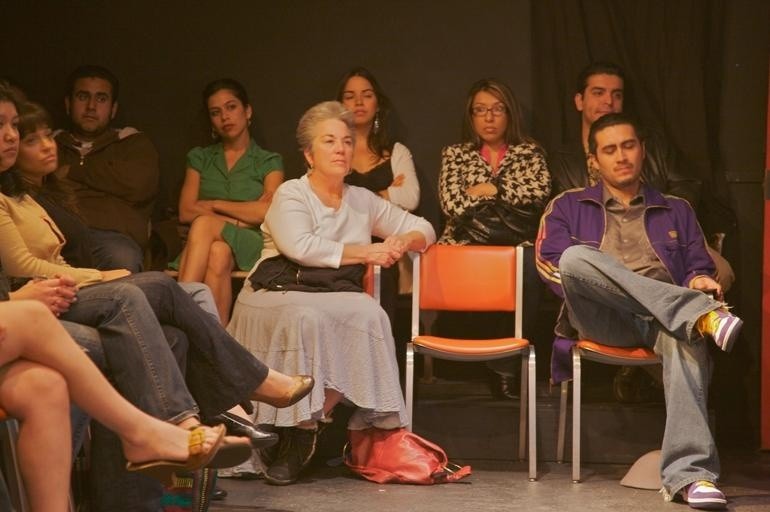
[694,303,744,354]
[677,479,728,510]
[265,427,316,485]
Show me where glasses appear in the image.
[471,104,505,116]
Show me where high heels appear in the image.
[240,374,315,414]
[126,411,279,487]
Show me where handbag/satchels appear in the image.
[344,426,471,484]
[462,199,545,245]
[249,252,366,292]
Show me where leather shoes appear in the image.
[502,373,520,399]
[613,366,638,403]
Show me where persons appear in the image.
[336,70,422,214]
[534,108,744,509]
[438,76,552,399]
[1,66,315,511]
[223,100,438,485]
[548,60,736,405]
